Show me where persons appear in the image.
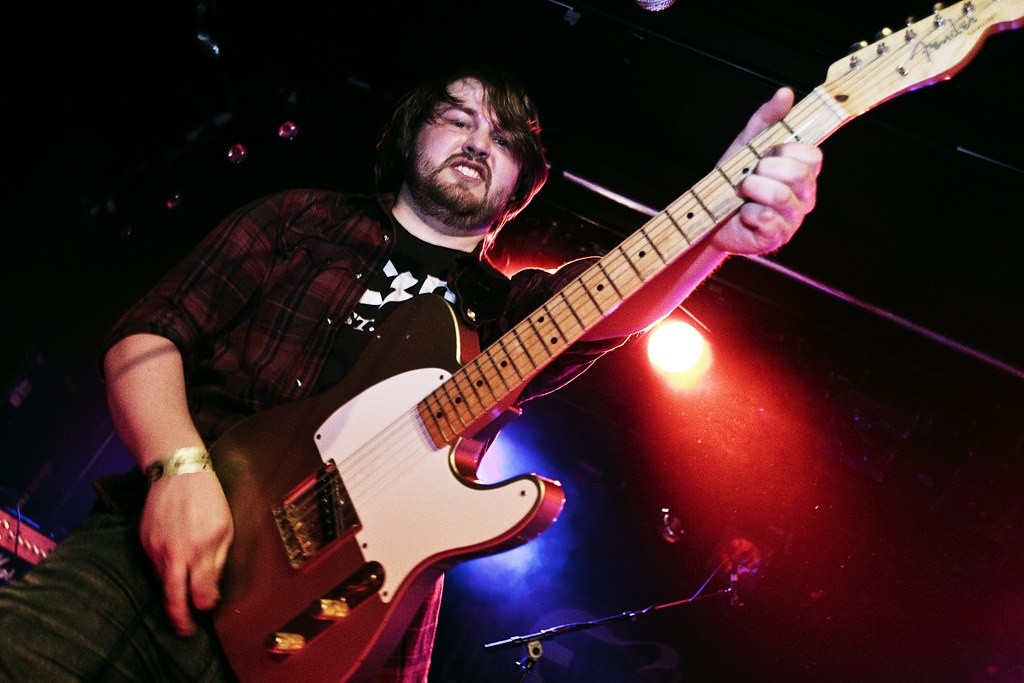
[0,50,824,683]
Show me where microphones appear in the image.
[637,0,675,12]
[717,539,760,584]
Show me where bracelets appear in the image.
[144,445,214,487]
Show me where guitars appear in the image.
[202,0,1024,681]
